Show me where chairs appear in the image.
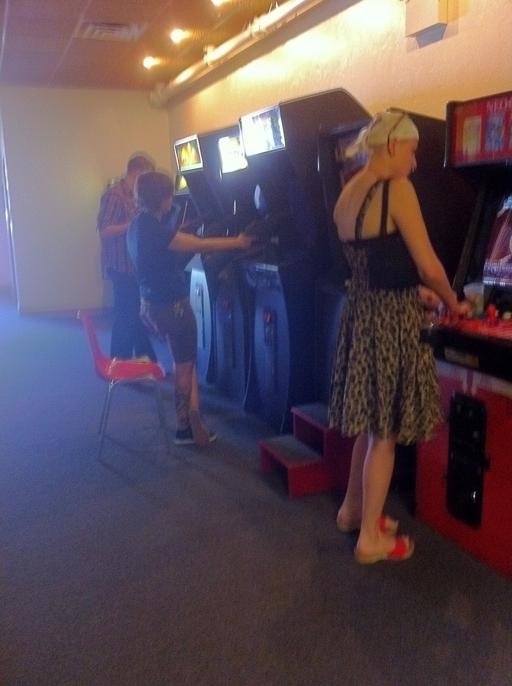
[74,307,173,462]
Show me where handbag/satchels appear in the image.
[367,235,420,290]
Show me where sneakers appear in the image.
[174,426,217,445]
[188,409,210,448]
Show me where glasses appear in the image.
[383,109,407,145]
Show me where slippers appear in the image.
[336,513,398,535]
[353,535,415,565]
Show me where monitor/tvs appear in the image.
[472,187,512,296]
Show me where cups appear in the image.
[485,107,505,152]
[463,282,482,313]
[461,114,482,158]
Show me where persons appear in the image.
[125,171,252,448]
[96,156,157,359]
[327,109,472,565]
